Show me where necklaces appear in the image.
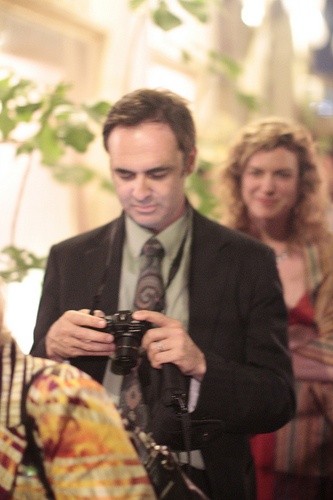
[273,237,293,265]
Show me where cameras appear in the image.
[93,310,154,369]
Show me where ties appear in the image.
[119,236,166,470]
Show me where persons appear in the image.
[0,287,158,500]
[26,87,298,500]
[220,115,333,500]
